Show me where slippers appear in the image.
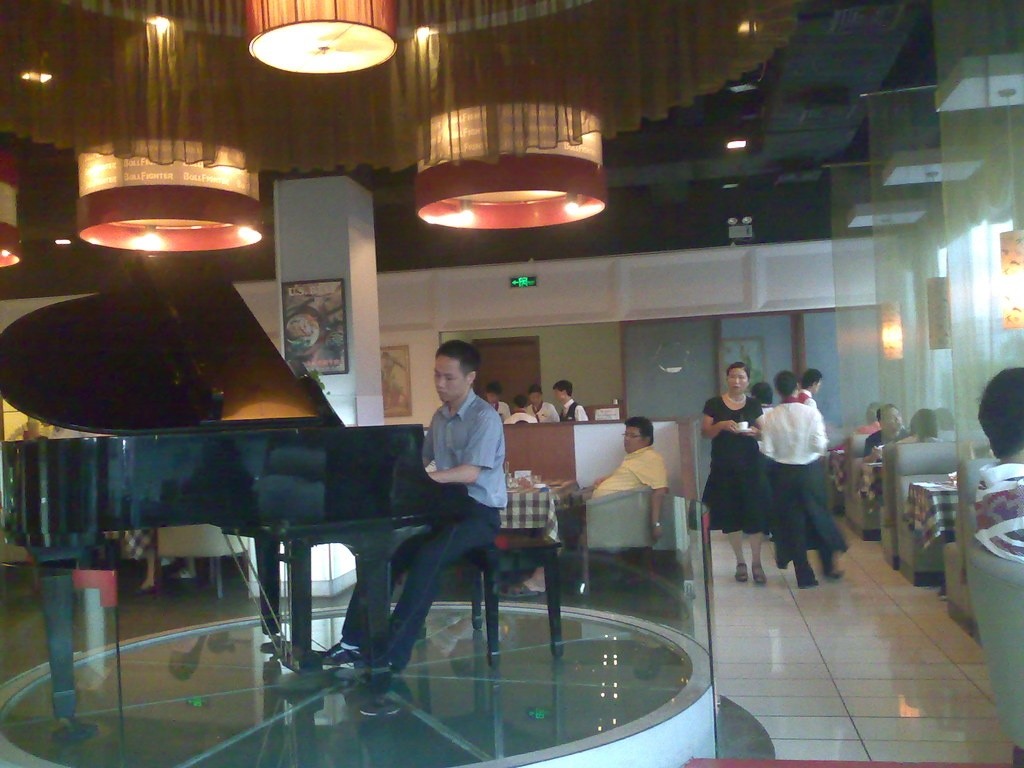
[499,581,539,598]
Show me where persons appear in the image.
[834,402,882,451]
[749,368,851,590]
[484,379,560,425]
[497,416,669,599]
[933,407,958,442]
[316,340,508,668]
[895,409,944,444]
[973,367,1024,563]
[701,362,771,584]
[864,404,912,496]
[553,380,589,422]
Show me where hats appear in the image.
[621,432,641,440]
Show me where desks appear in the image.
[829,449,850,494]
[904,481,958,550]
[852,458,883,513]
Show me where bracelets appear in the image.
[652,522,663,527]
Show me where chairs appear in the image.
[156,523,248,599]
[569,486,657,616]
[503,478,579,594]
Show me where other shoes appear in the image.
[800,577,819,589]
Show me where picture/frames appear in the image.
[717,338,767,398]
[281,279,349,377]
[379,343,410,418]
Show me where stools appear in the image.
[471,531,564,667]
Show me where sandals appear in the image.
[735,563,749,582]
[752,563,767,583]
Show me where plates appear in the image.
[737,429,752,433]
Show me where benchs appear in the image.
[826,426,1000,615]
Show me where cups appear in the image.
[532,475,542,486]
[504,473,511,490]
[737,421,748,429]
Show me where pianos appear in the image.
[1,274,473,726]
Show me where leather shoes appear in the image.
[315,641,356,666]
[367,654,406,672]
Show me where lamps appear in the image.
[413,98,608,229]
[727,215,753,238]
[993,105,1024,273]
[1,148,21,267]
[1002,273,1024,332]
[881,301,904,360]
[73,129,265,253]
[245,0,397,74]
[928,276,951,349]
